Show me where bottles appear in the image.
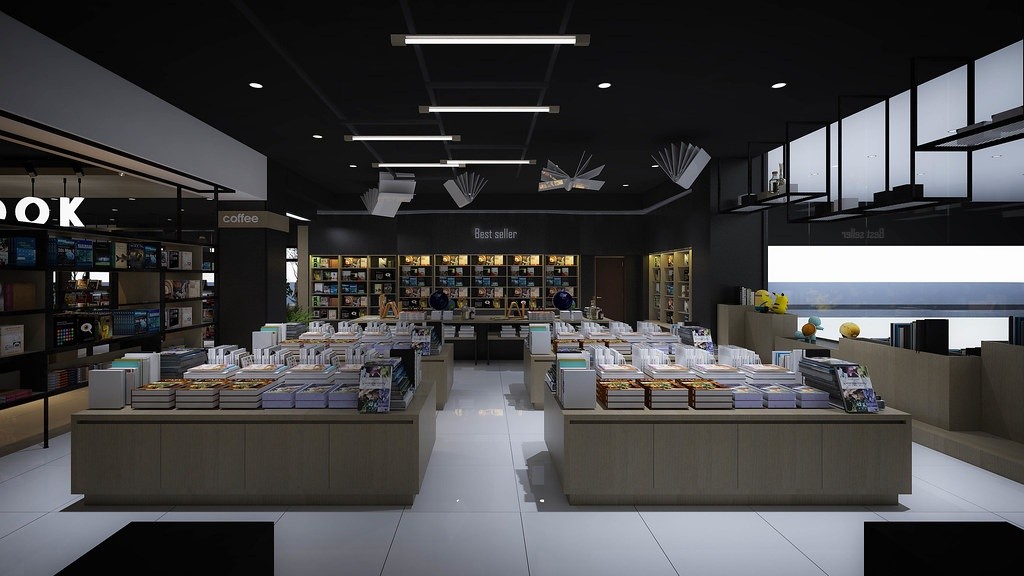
[768,164,786,193]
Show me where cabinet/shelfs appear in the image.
[308,253,582,322]
[0,221,219,450]
[543,384,912,506]
[69,380,437,508]
[648,247,706,332]
[418,344,454,411]
[523,338,556,411]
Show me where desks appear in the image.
[352,315,616,360]
[444,334,478,365]
[487,332,526,365]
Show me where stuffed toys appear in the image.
[755,290,770,313]
[794,316,824,344]
[762,291,788,314]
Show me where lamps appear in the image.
[440,159,536,165]
[344,135,461,141]
[371,163,466,168]
[390,33,591,47]
[419,106,559,113]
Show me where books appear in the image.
[525,321,886,413]
[0,231,214,403]
[652,254,689,325]
[311,255,574,338]
[87,321,445,414]
[1009,315,1024,346]
[739,285,754,306]
[889,319,949,354]
